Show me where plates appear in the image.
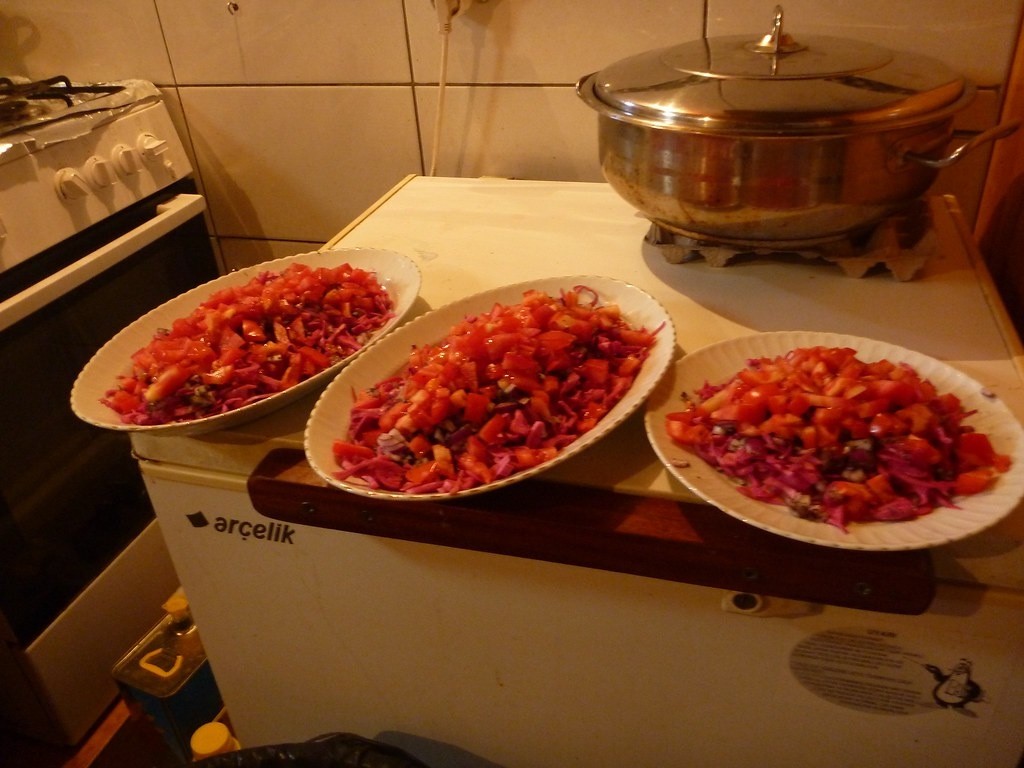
[70,248,422,437]
[303,275,676,501]
[645,331,1023,552]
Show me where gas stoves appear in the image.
[0,72,195,303]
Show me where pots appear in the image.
[576,5,1018,249]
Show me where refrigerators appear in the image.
[124,172,1024,768]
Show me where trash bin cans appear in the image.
[181,731,427,768]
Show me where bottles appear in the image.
[190,721,242,768]
